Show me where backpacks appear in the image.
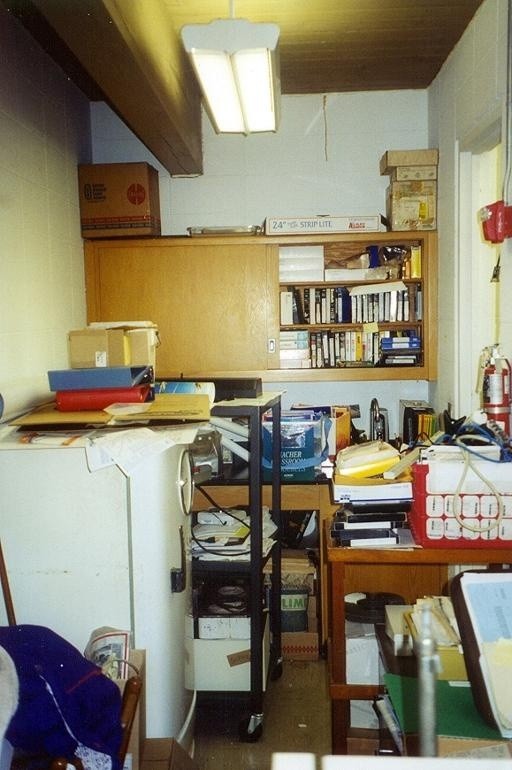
[0,625,124,766]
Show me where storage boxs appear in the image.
[75,162,162,238]
[378,148,440,232]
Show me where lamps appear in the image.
[178,1,282,138]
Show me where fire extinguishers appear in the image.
[475,343,512,437]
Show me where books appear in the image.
[190,505,281,563]
[329,499,417,552]
[401,250,411,280]
[323,258,388,282]
[54,383,152,413]
[281,283,424,370]
[260,397,442,482]
[410,245,422,279]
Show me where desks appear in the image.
[322,517,512,754]
